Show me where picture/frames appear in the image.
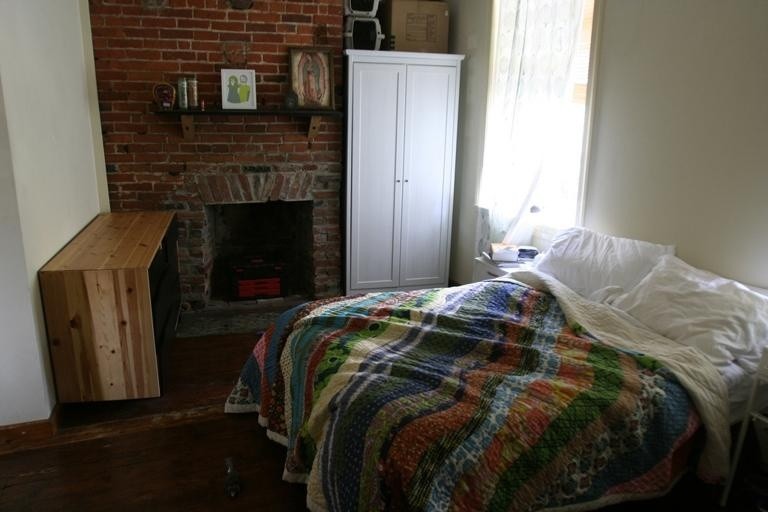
[287,45,337,111]
[219,66,259,112]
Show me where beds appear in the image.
[274,265,767,512]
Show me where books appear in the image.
[482,242,517,267]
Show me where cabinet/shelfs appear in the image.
[340,49,466,297]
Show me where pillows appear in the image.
[533,225,767,394]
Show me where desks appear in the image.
[38,211,186,404]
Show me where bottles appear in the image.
[176,77,199,111]
[224,456,241,498]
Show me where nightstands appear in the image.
[473,256,536,282]
